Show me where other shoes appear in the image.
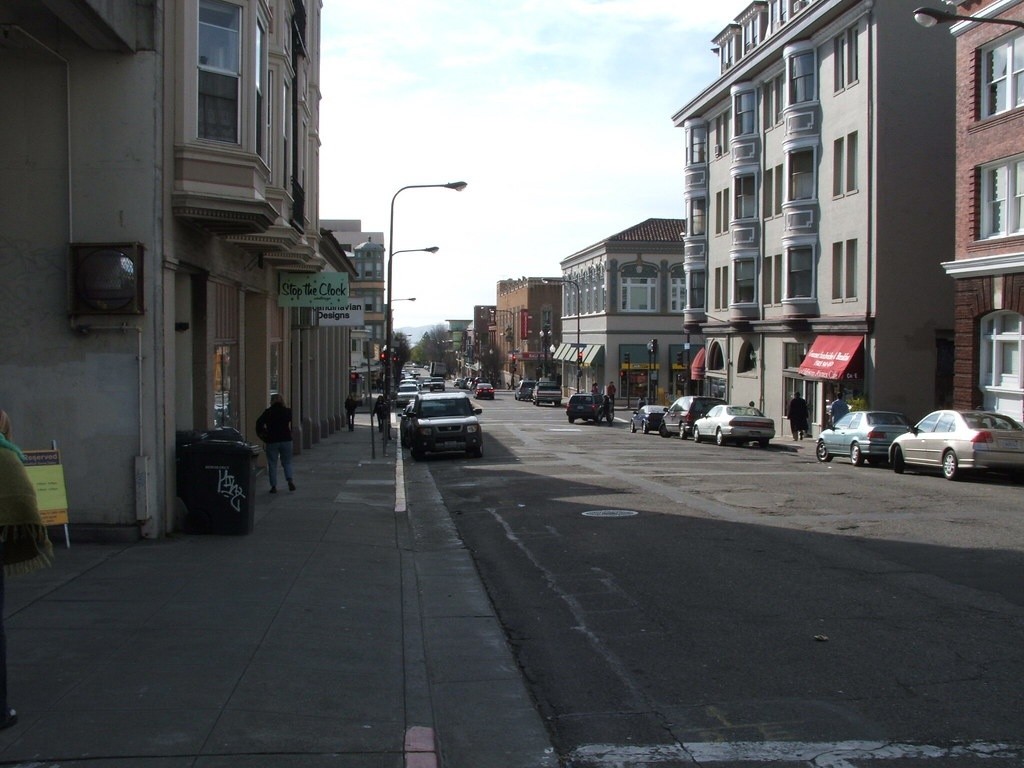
[268,486,277,493]
[288,479,295,491]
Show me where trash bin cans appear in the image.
[180,440,262,535]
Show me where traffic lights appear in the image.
[676,351,683,366]
[624,352,630,363]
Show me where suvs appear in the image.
[658,395,729,440]
[514,379,537,401]
[407,390,484,463]
[528,381,562,407]
[565,393,615,424]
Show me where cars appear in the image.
[888,408,1024,480]
[399,362,489,449]
[692,405,776,447]
[816,410,918,468]
[473,382,494,400]
[630,405,670,434]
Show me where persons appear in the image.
[256,394,296,493]
[749,401,755,407]
[602,395,613,427]
[607,381,616,407]
[0,409,54,731]
[828,393,849,429]
[344,395,357,432]
[638,394,646,410]
[787,391,809,442]
[591,383,599,395]
[373,396,385,433]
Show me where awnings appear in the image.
[798,335,865,382]
[691,347,705,381]
[553,343,604,366]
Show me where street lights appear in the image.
[384,246,440,443]
[488,308,516,390]
[541,278,580,393]
[382,181,469,440]
[549,343,556,381]
[540,329,552,378]
